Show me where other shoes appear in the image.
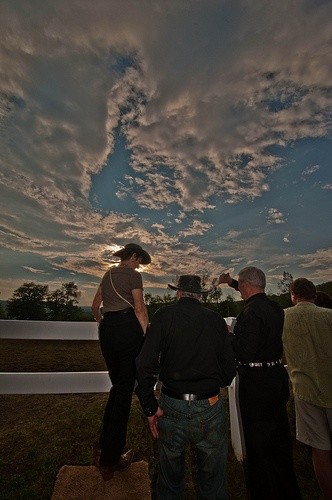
[102,447,134,481]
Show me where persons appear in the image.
[313,291,332,309]
[135,275,235,500]
[92,244,151,480]
[282,278,332,500]
[218,266,302,500]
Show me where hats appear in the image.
[168,275,212,293]
[113,244,151,264]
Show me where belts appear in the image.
[161,387,220,401]
[250,359,283,368]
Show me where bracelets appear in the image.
[96,316,102,322]
